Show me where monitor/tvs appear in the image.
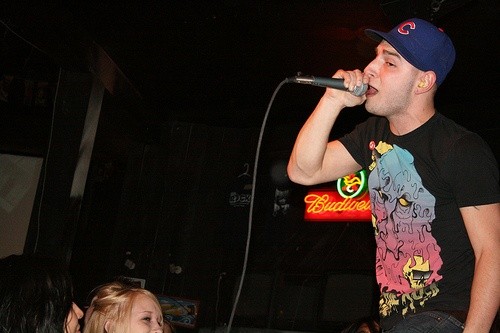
[233,275,270,317]
[320,276,374,322]
[275,284,319,319]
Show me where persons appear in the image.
[284,14,500,333]
[82,282,164,333]
[0,253,85,333]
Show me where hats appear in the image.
[365,19,456,89]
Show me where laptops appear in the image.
[154,294,202,333]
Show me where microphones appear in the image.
[285,73,368,96]
